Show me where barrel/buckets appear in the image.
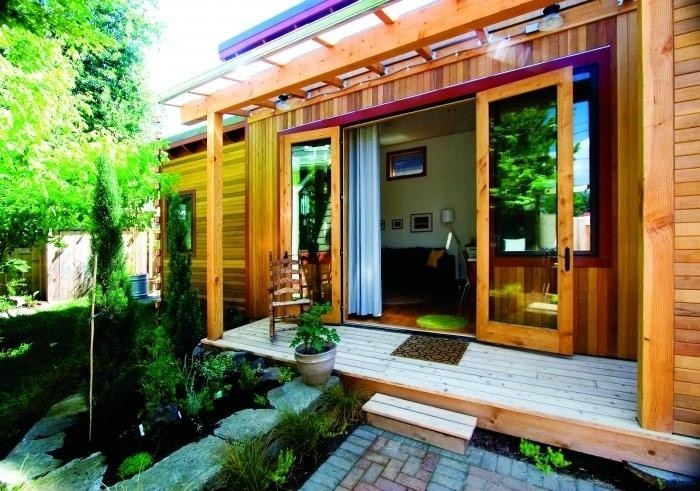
[130,274,147,299]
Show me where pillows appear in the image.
[381,246,454,270]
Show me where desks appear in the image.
[467,256,477,285]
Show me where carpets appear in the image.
[414,312,469,329]
[391,334,469,366]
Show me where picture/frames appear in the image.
[381,213,433,232]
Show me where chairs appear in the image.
[459,250,470,307]
[268,250,313,341]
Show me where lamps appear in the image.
[276,94,292,110]
[441,209,461,251]
[538,5,564,31]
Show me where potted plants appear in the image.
[290,303,341,386]
